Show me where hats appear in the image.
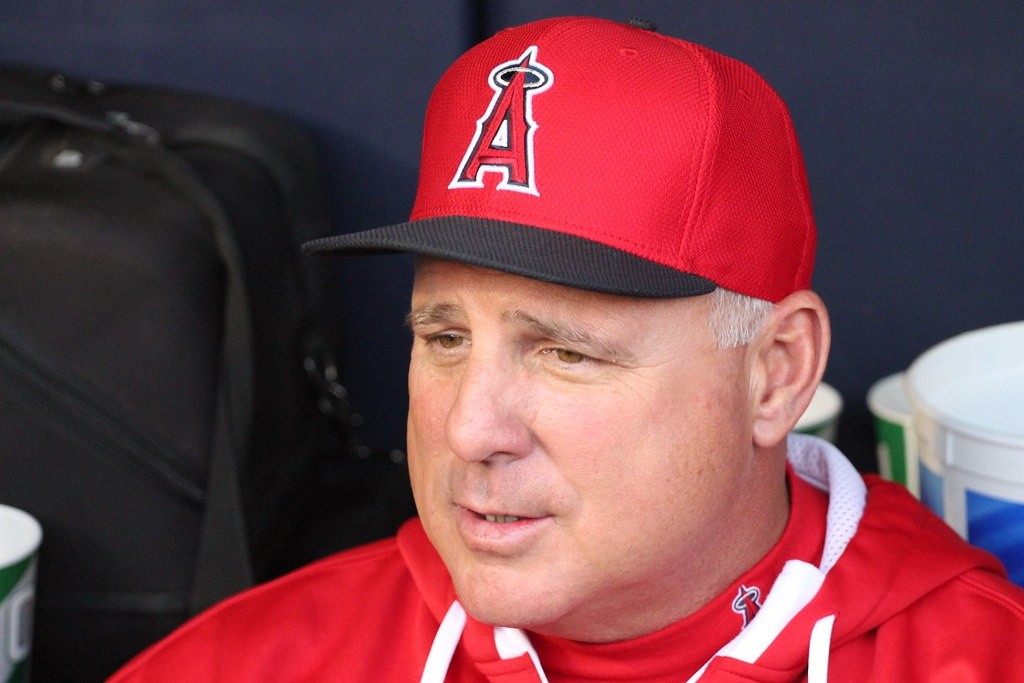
[300,16,816,305]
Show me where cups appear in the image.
[790,381,844,445]
[867,371,920,500]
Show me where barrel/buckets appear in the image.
[906,321,1024,589]
[0,503,43,682]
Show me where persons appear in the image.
[111,15,1024,683]
